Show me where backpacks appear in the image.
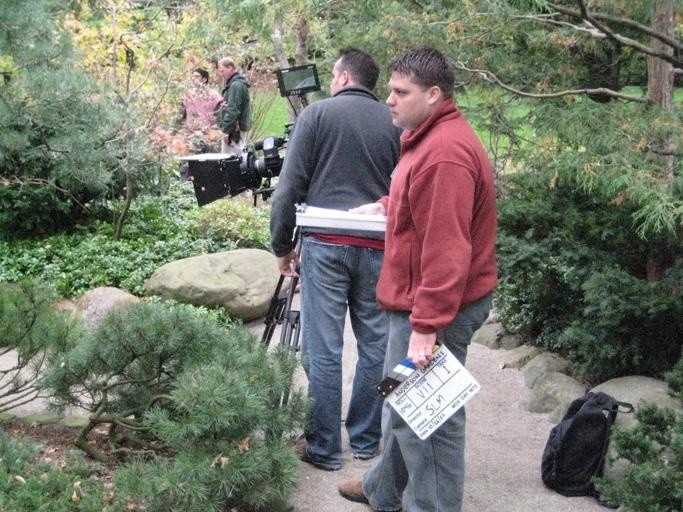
[540,392,633,497]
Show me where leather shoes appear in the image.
[338,480,372,504]
[295,440,334,472]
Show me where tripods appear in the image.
[259,227,302,445]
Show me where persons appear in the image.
[271,46,403,470]
[339,48,497,511]
[215,57,253,154]
[170,67,239,182]
